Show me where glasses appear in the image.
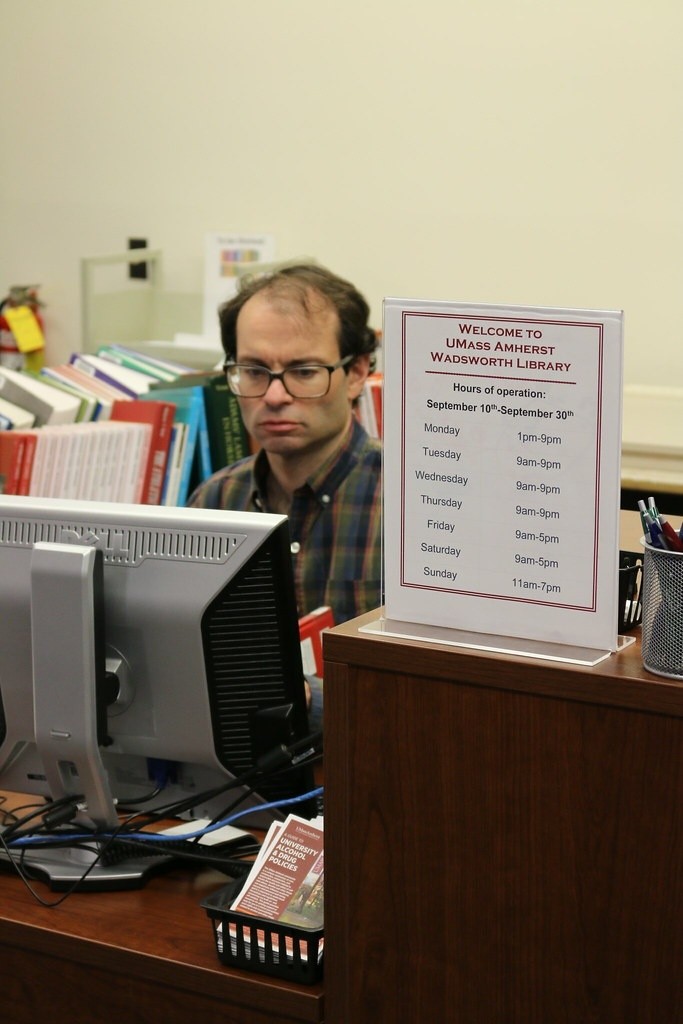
[221,351,360,399]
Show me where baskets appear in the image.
[638,529,683,681]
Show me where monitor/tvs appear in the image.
[0,494,314,891]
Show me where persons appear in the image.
[182,258,383,770]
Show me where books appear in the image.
[0,342,385,506]
[296,603,337,679]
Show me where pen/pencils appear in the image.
[644,513,668,551]
[648,497,659,520]
[657,514,683,553]
[637,500,654,547]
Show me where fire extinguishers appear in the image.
[0,285,48,372]
